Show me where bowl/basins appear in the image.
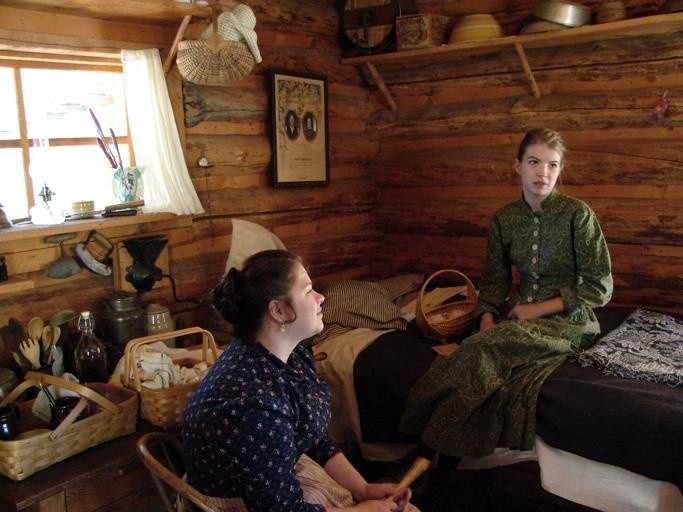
[532,0,593,28]
[446,12,503,48]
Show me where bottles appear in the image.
[102,292,145,367]
[46,396,89,431]
[73,310,107,381]
[145,301,177,348]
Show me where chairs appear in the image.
[136,432,249,511]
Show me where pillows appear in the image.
[319,271,426,332]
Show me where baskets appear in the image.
[0,372,140,482]
[116,325,225,428]
[413,268,480,341]
[176,1,256,86]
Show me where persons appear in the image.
[383,128,616,511]
[302,116,316,142]
[173,246,424,512]
[284,112,299,140]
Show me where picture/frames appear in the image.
[269,68,329,191]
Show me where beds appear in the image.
[309,263,683,512]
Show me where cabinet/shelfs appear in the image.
[0,429,178,512]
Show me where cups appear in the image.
[0,404,22,441]
[72,199,96,218]
